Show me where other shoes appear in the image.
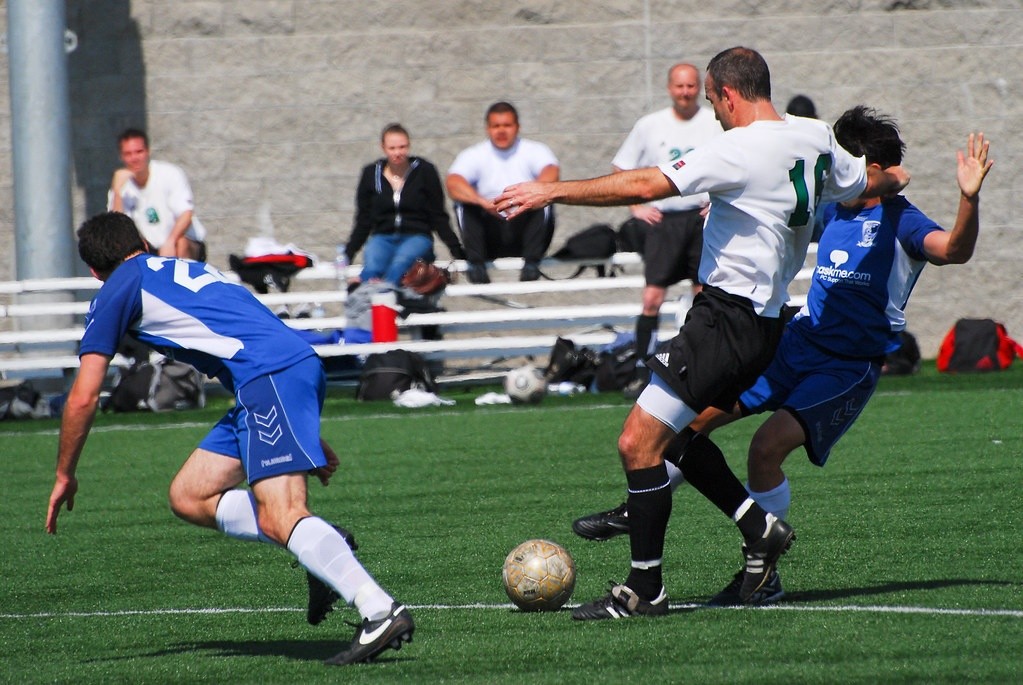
[621,376,647,399]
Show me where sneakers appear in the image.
[292,525,357,626]
[571,502,629,542]
[570,581,669,621]
[738,513,796,601]
[707,569,786,606]
[324,602,415,667]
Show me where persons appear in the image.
[45,210,415,666]
[573,105,993,609]
[333,123,467,289]
[610,64,727,397]
[493,45,911,620]
[445,103,561,284]
[786,96,819,118]
[107,128,207,263]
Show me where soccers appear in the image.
[502,365,547,403]
[501,539,576,612]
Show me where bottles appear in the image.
[335,245,347,294]
[308,301,326,319]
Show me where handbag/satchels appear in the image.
[356,347,433,400]
[402,260,452,295]
[942,316,1023,372]
[0,353,205,417]
[545,331,633,389]
[551,217,617,260]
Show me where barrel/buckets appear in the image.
[370,291,404,342]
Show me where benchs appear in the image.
[0,241,819,372]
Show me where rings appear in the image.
[510,199,513,207]
